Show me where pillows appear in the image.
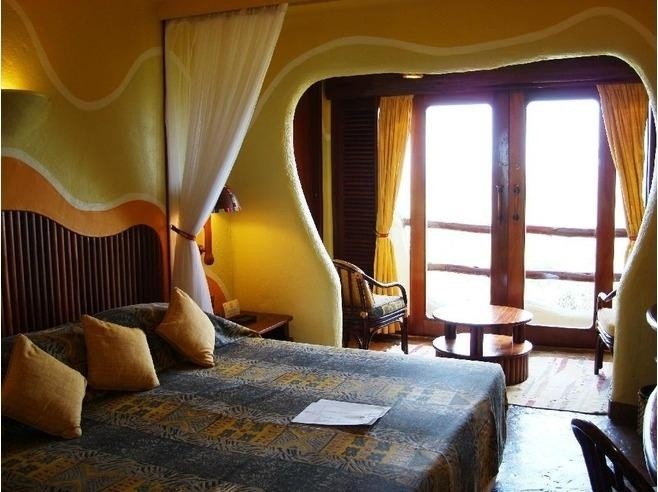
[155,288,216,368]
[2,332,89,440]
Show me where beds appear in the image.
[1,156,509,491]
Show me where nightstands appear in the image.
[214,310,294,340]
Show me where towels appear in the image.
[81,315,160,391]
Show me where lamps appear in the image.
[203,183,241,265]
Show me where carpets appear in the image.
[406,337,613,413]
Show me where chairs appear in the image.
[592,289,618,374]
[570,419,652,492]
[333,258,409,352]
